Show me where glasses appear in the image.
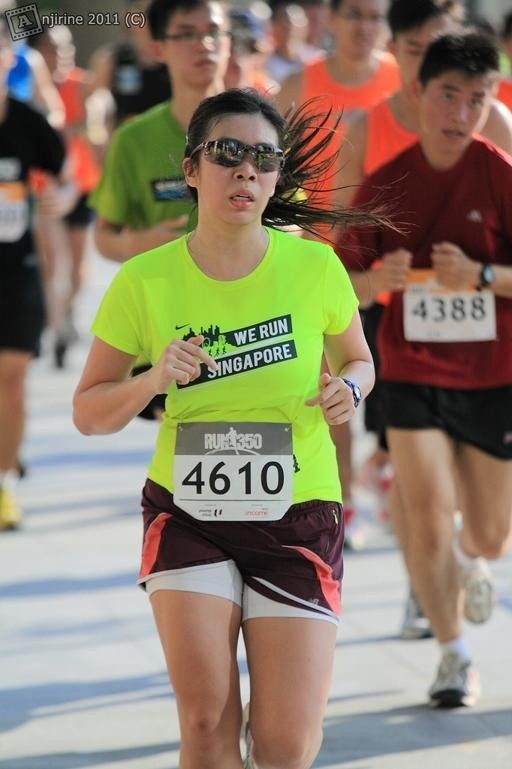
[163,29,233,42]
[189,139,285,172]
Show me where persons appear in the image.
[327,32,510,709]
[71,86,377,767]
[2,0,510,642]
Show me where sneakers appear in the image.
[404,536,495,708]
